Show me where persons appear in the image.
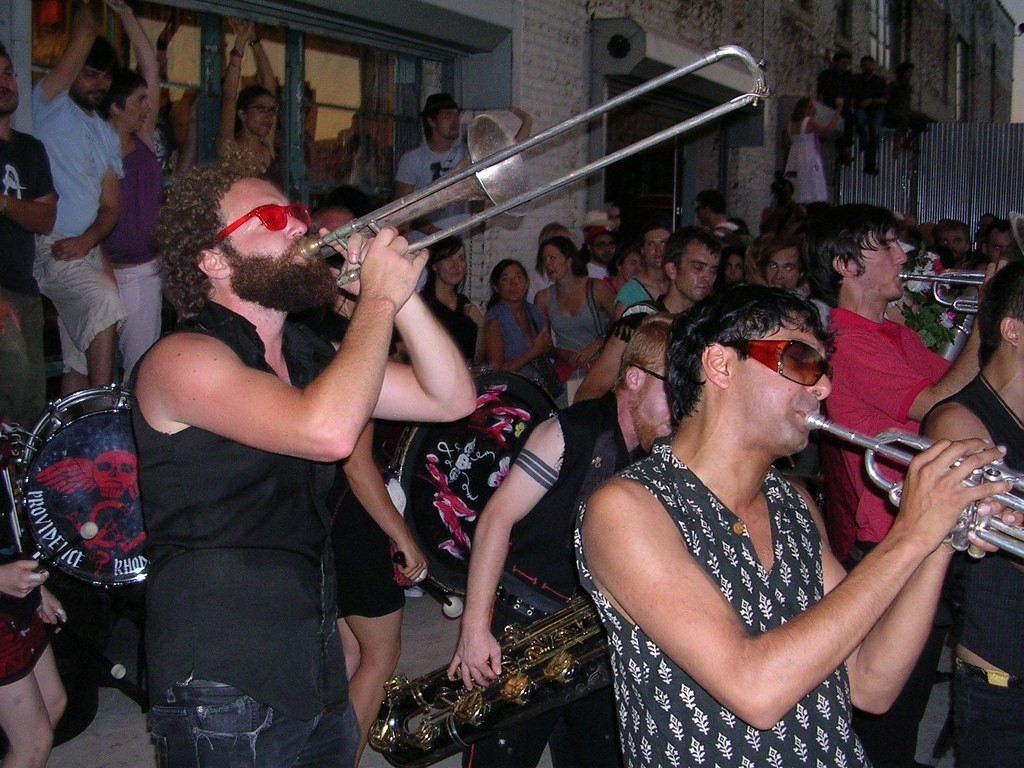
[345,133,399,185]
[0,0,1024,768]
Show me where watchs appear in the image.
[249,38,259,47]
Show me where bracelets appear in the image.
[230,47,243,59]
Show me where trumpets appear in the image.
[801,408,1024,559]
[898,271,986,313]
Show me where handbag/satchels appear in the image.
[540,364,564,399]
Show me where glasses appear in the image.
[210,201,314,246]
[989,240,1014,252]
[726,337,833,387]
[249,104,277,115]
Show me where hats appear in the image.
[61,34,122,75]
[417,92,458,114]
[578,209,616,232]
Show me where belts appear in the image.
[852,536,877,554]
[494,587,548,621]
[953,660,1024,690]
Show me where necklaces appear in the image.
[243,134,266,166]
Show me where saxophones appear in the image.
[368,596,612,768]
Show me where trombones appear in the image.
[297,44,774,288]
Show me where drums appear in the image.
[17,382,151,589]
[387,365,561,597]
